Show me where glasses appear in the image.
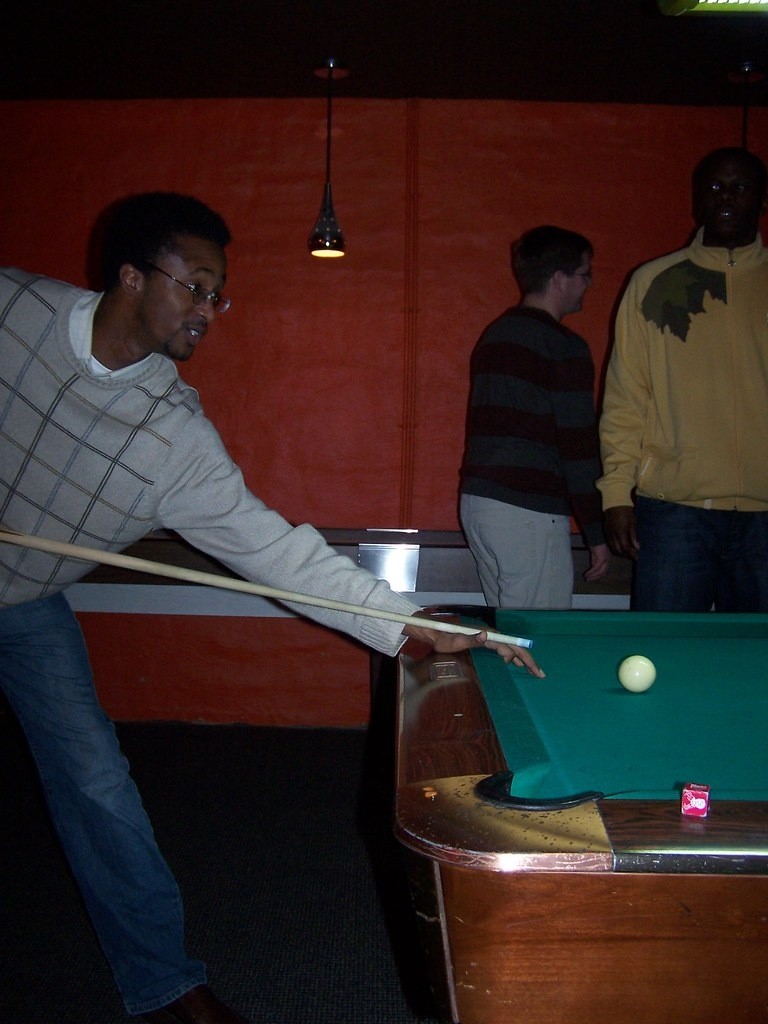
[566,270,593,285]
[143,260,231,313]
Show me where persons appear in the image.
[458,223,612,610]
[0,188,549,1024]
[593,145,768,612]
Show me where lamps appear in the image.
[659,1,766,18]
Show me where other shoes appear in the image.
[140,984,253,1024]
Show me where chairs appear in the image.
[309,5,346,260]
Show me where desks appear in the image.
[67,525,635,724]
[396,611,768,1023]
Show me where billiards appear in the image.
[617,655,656,693]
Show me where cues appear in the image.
[0,530,535,650]
